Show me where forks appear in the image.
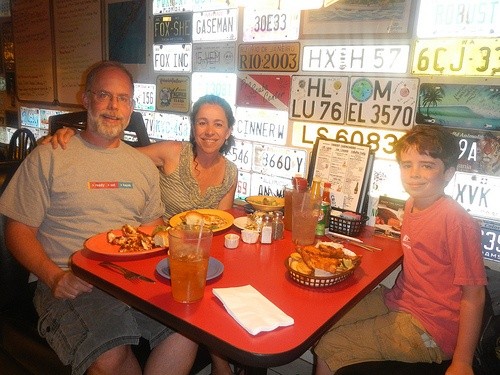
[99,262,143,284]
[326,234,372,251]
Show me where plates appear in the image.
[83,226,169,256]
[169,208,234,232]
[155,255,224,280]
[233,216,262,232]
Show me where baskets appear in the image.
[329,207,370,237]
[285,247,360,287]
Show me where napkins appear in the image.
[212,284,295,336]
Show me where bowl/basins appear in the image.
[245,196,285,213]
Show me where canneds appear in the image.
[314,201,330,236]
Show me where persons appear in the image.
[310,125,488,375]
[39,94,238,375]
[0,62,199,375]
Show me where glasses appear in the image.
[88,89,131,104]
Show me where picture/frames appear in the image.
[299,0,420,40]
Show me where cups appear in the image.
[224,233,239,248]
[241,229,259,244]
[291,192,321,246]
[168,224,213,303]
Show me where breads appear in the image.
[290,253,354,277]
[153,231,169,247]
[183,211,221,226]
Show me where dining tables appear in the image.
[69,204,403,375]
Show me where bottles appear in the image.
[255,211,284,244]
[284,176,331,238]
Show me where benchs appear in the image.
[0,160,266,375]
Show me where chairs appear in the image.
[48,111,151,148]
[334,266,500,375]
[0,128,39,194]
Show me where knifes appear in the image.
[105,261,157,283]
